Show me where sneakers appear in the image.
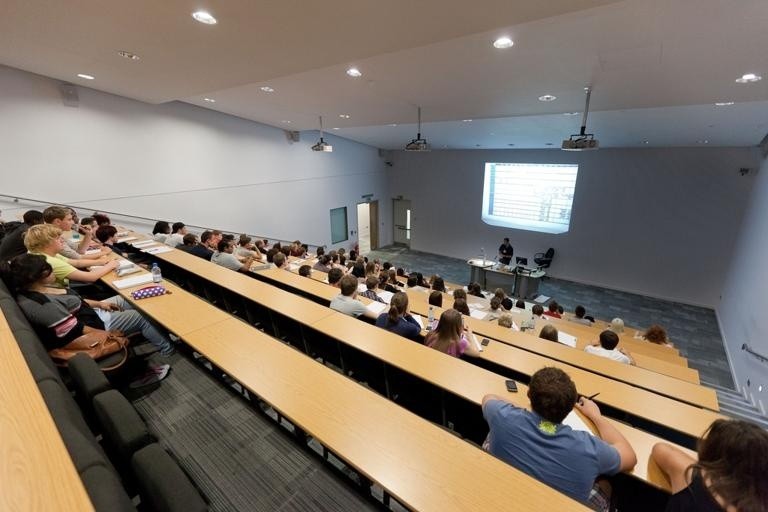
[161,338,181,357]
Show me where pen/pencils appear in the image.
[580,392,601,407]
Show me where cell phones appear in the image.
[426,325,432,330]
[506,379,518,392]
[481,339,489,346]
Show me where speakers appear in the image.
[287,130,299,142]
[379,149,385,158]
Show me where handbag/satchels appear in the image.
[49,331,131,371]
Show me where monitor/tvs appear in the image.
[516,257,527,272]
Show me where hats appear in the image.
[171,223,184,233]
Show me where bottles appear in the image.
[72,224,79,241]
[151,263,161,283]
[428,307,433,322]
[529,316,535,335]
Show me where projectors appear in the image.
[562,140,599,151]
[404,143,432,153]
[312,145,333,152]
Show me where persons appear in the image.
[498,238,514,265]
[634,324,673,349]
[425,308,472,357]
[365,258,525,330]
[607,318,627,336]
[253,238,368,287]
[651,418,767,511]
[538,324,559,342]
[0,204,164,390]
[480,365,638,512]
[583,330,637,367]
[329,274,377,319]
[152,221,262,273]
[530,299,596,327]
[375,292,421,336]
